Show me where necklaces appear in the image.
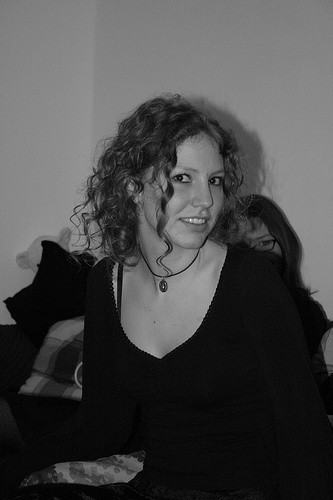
[138,240,201,292]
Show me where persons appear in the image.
[224,194,303,273]
[2,96,333,500]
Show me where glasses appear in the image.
[248,238,277,251]
